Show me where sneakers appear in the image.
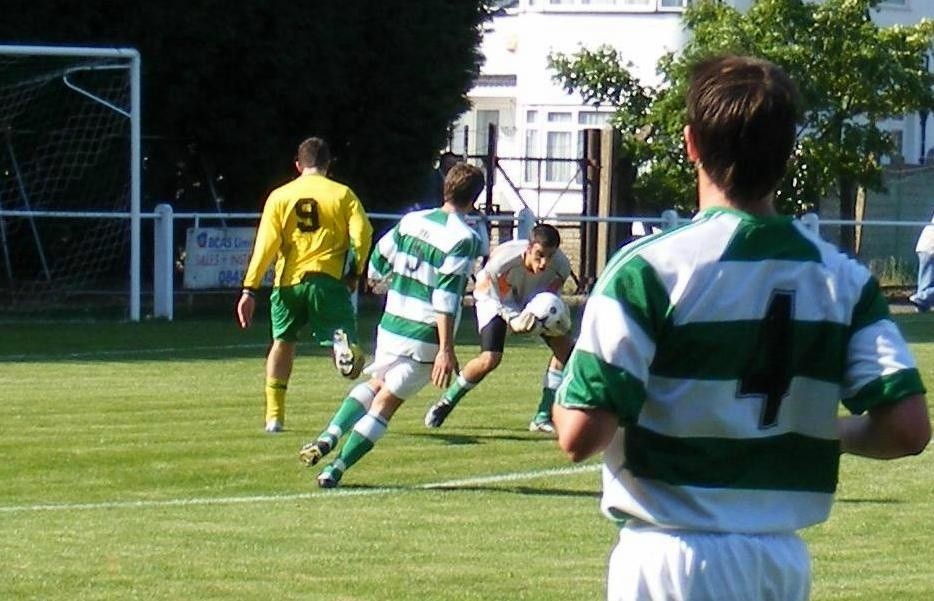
[425,398,453,430]
[528,412,558,436]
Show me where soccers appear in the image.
[524,291,566,332]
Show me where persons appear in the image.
[419,152,459,210]
[551,59,934,601]
[425,224,577,433]
[299,164,486,488]
[909,214,934,313]
[237,137,374,432]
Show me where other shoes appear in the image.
[318,466,343,488]
[910,294,931,312]
[333,328,354,375]
[299,441,330,466]
[265,408,286,432]
